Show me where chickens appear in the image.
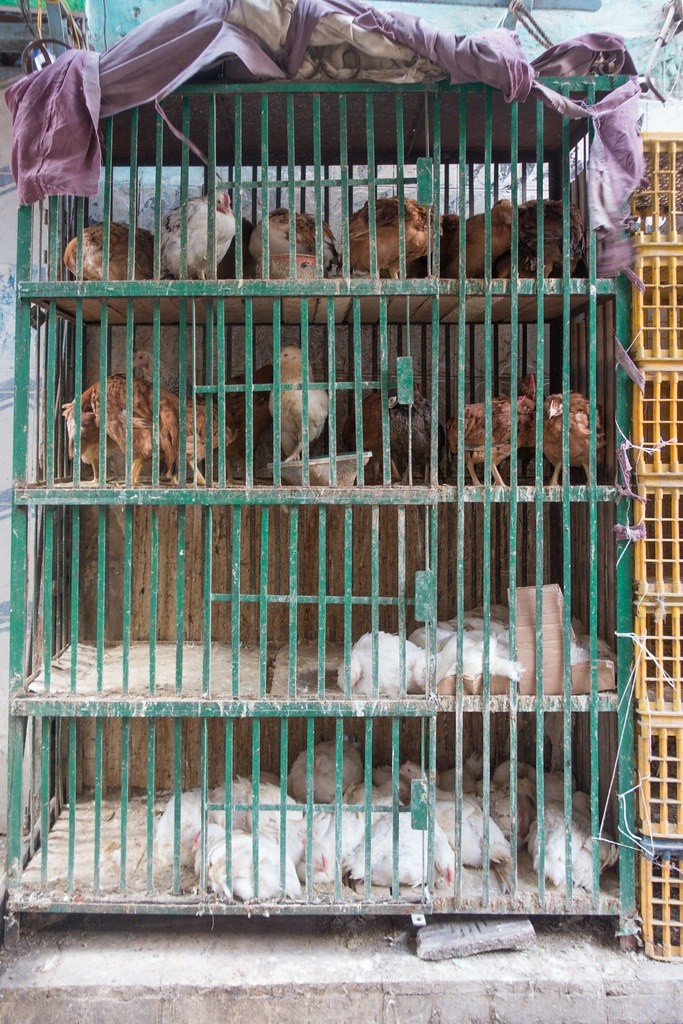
[154,739,619,900]
[63,190,587,280]
[61,346,608,488]
[337,605,619,697]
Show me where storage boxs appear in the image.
[629,129,682,962]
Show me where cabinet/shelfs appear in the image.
[3,75,636,964]
